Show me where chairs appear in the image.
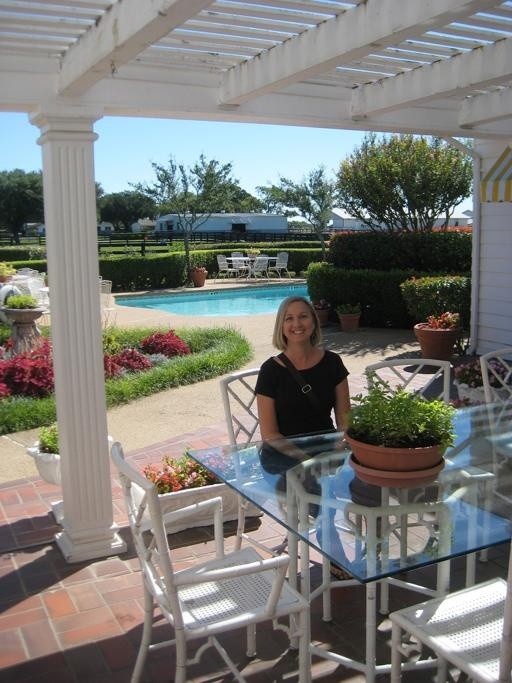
[214,252,291,284]
[14,268,116,328]
[216,365,309,552]
[366,358,451,409]
[387,570,511,683]
[480,350,512,404]
[109,441,314,683]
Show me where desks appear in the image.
[186,402,511,683]
[0,274,44,301]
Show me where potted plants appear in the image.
[190,268,208,287]
[27,422,112,526]
[341,383,456,490]
[0,263,16,282]
[312,302,328,327]
[335,304,361,330]
[0,294,46,322]
[245,249,260,258]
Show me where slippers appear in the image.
[316,530,356,581]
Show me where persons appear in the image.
[253,296,381,580]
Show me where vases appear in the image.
[414,324,462,360]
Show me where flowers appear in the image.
[420,311,458,329]
